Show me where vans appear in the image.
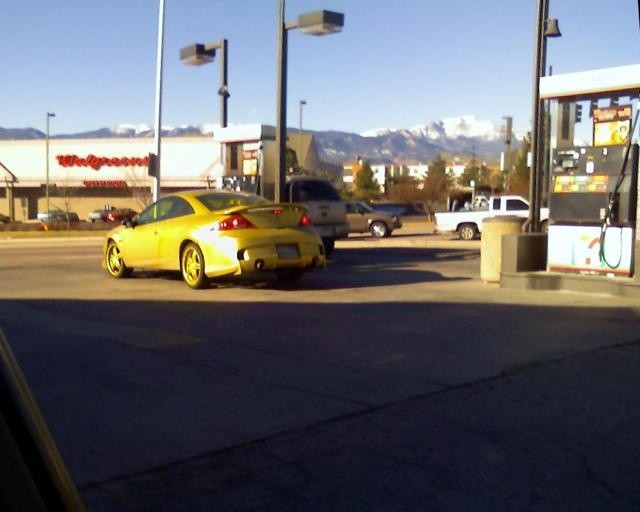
[284,173,353,257]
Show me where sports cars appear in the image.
[101,186,331,291]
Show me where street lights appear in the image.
[271,9,346,208]
[45,109,58,213]
[174,37,230,136]
[299,98,308,170]
[518,0,563,236]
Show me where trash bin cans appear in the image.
[480,215,529,283]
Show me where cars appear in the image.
[343,200,402,238]
[35,206,144,223]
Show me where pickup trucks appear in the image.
[432,194,549,242]
